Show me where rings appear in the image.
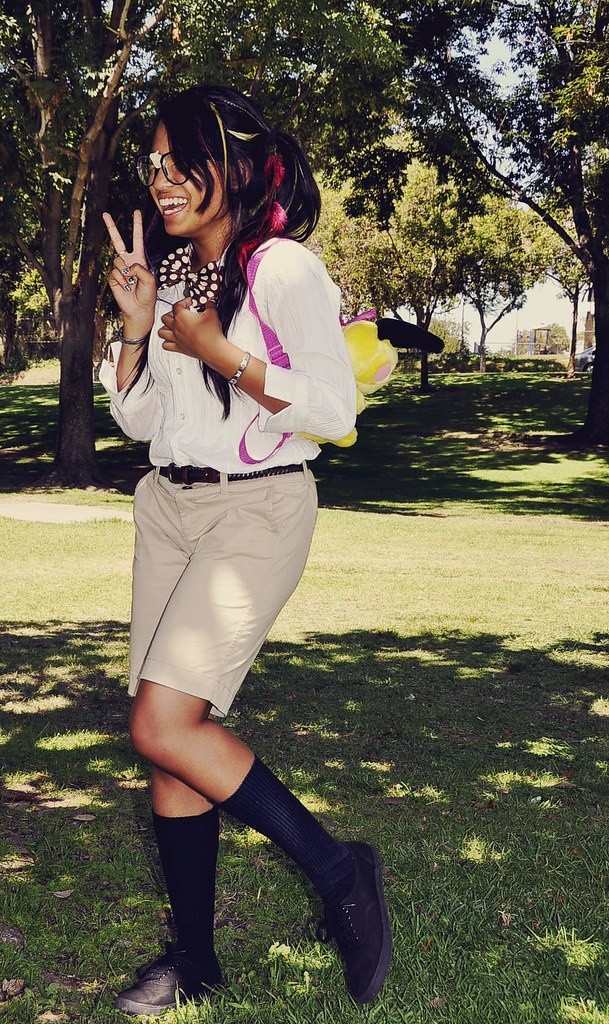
[113,250,127,258]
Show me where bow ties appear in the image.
[157,246,222,308]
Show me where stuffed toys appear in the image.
[296,318,444,448]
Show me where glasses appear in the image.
[136,151,223,187]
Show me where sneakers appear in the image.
[316,840,392,1003]
[116,941,224,1017]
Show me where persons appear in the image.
[100,86,392,1017]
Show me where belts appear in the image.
[155,462,312,485]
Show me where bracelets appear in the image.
[117,326,144,345]
[229,351,251,385]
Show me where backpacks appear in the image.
[239,238,445,465]
